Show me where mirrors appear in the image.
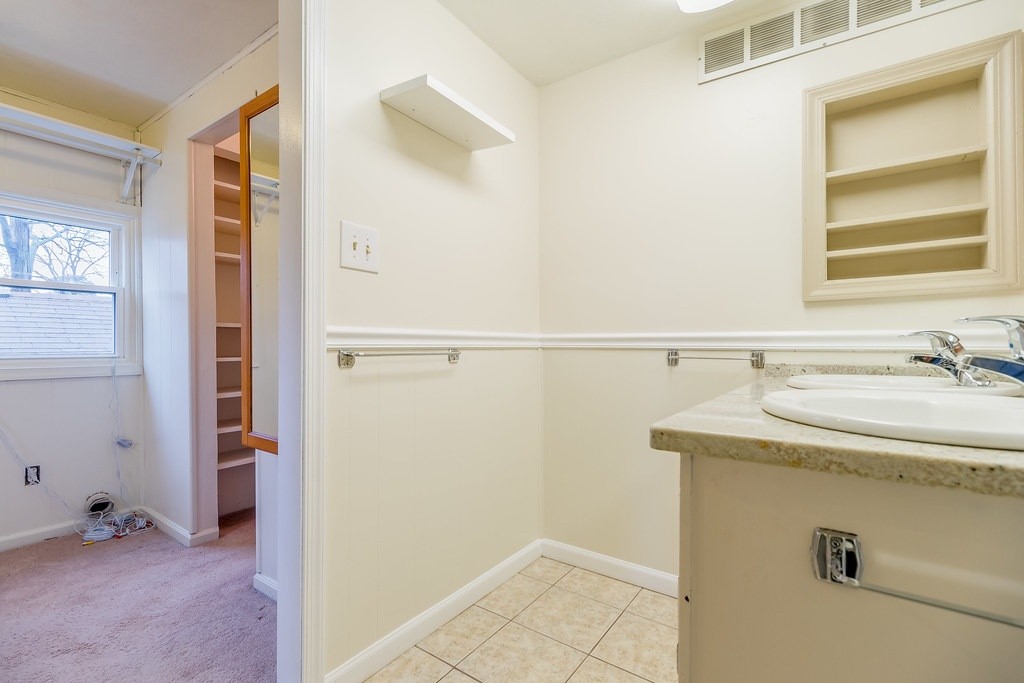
[241,83,286,455]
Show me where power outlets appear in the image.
[25,465,40,486]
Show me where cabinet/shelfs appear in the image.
[802,25,1023,304]
[214,181,256,472]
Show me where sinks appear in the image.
[755,387,1024,452]
[783,372,1024,397]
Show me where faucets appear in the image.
[896,328,998,388]
[953,312,1024,388]
